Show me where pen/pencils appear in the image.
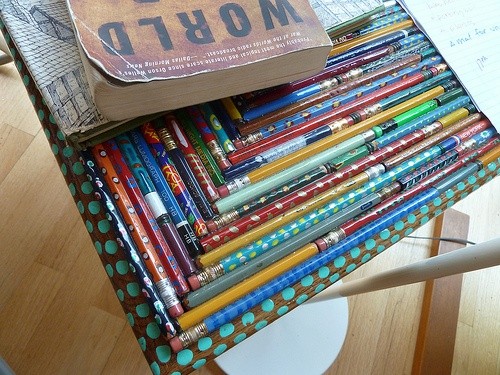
[69,0,500,359]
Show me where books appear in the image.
[66,1,334,120]
[0,0,386,151]
